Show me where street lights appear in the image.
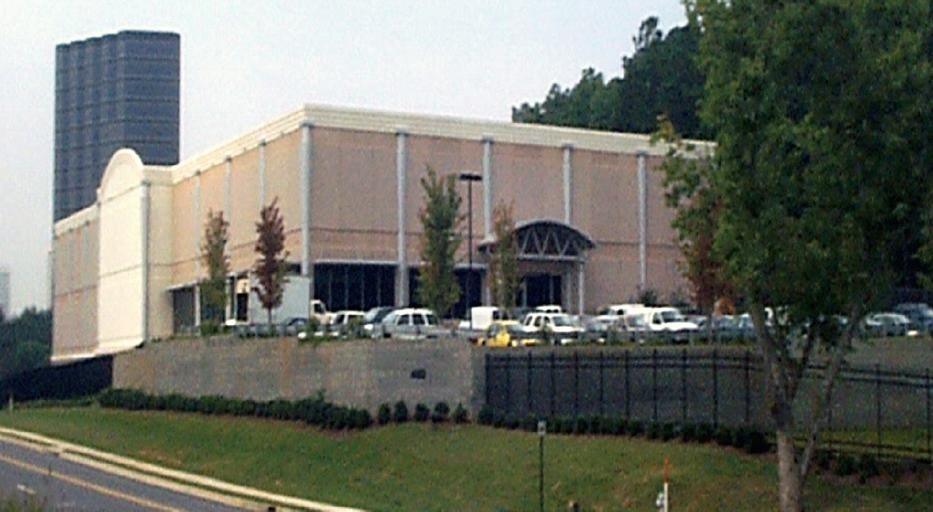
[459,172,483,317]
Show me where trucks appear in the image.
[226,277,331,337]
